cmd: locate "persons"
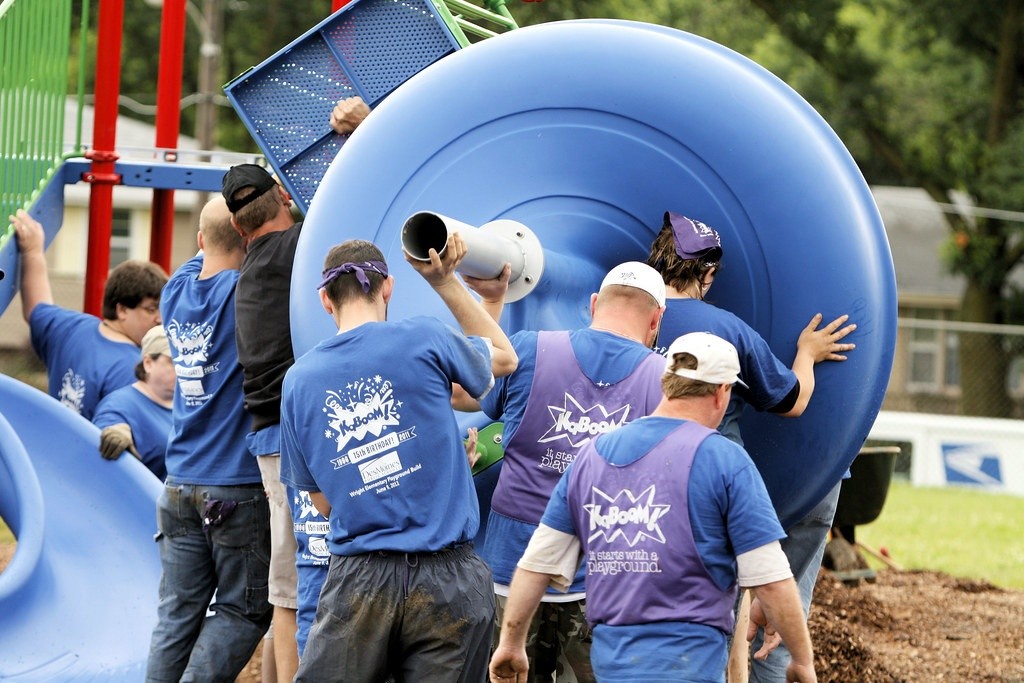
[745,478,842,683]
[294,423,485,670]
[451,260,677,683]
[650,210,857,459]
[272,231,520,683]
[145,197,275,683]
[91,325,176,488]
[489,331,817,683]
[10,207,173,429]
[220,96,371,683]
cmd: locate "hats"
[665,331,749,389]
[222,164,279,214]
[140,325,172,358]
[598,261,666,308]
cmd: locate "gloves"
[99,423,142,461]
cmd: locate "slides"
[0,374,165,683]
